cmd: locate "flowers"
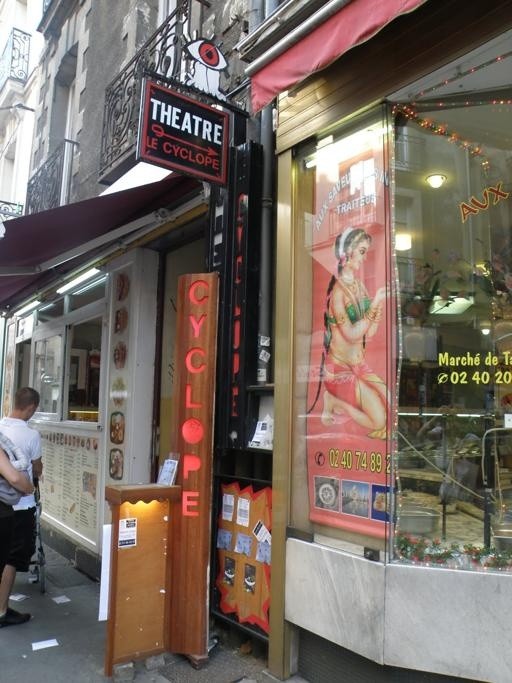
[398,530,512,570]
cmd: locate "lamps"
[405,291,477,316]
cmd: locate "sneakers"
[0,607,31,629]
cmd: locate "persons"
[417,405,482,504]
[307,228,396,431]
[0,387,43,627]
